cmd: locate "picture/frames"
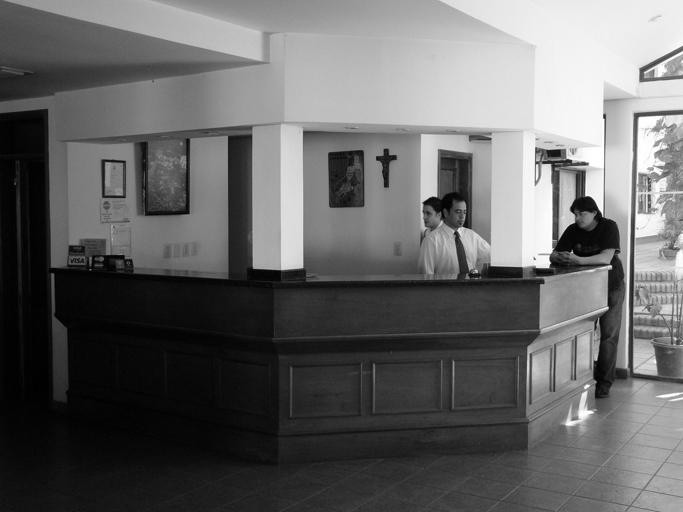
[101,159,126,198]
[141,139,190,215]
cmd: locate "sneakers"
[595,380,611,398]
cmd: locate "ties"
[454,230,470,274]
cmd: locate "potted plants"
[636,273,683,378]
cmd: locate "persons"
[417,192,491,274]
[421,195,443,241]
[549,196,626,398]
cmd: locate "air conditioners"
[543,148,581,162]
